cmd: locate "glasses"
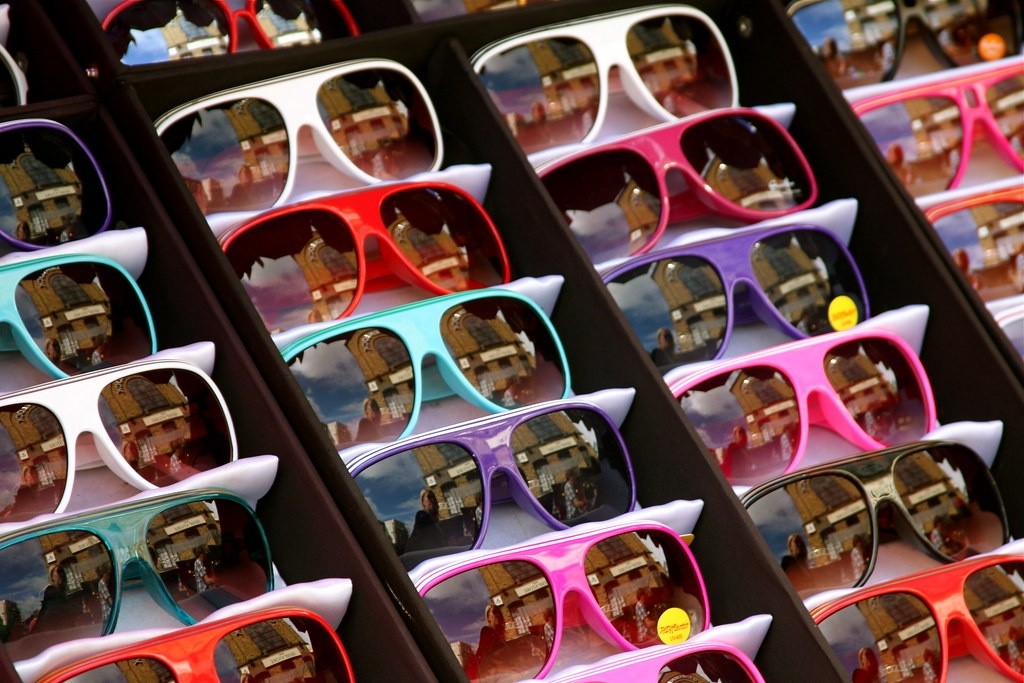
[153,57,444,214]
[0,40,31,111]
[0,251,158,380]
[0,486,274,662]
[409,518,710,683]
[1,119,112,248]
[34,606,356,682]
[217,182,510,337]
[271,288,571,451]
[344,399,638,573]
[550,638,766,683]
[99,0,362,65]
[0,359,240,521]
[810,556,1023,683]
[405,0,1024,598]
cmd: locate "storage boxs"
[0,0,1023,683]
